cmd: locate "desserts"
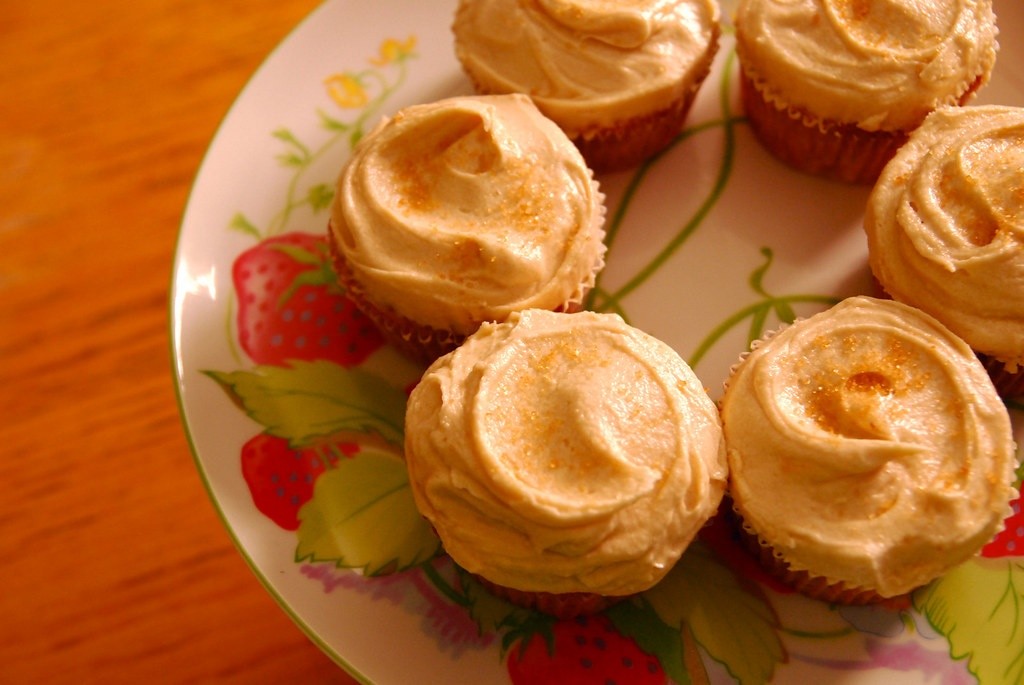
[324,0,1023,619]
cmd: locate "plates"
[167,0,1024,685]
